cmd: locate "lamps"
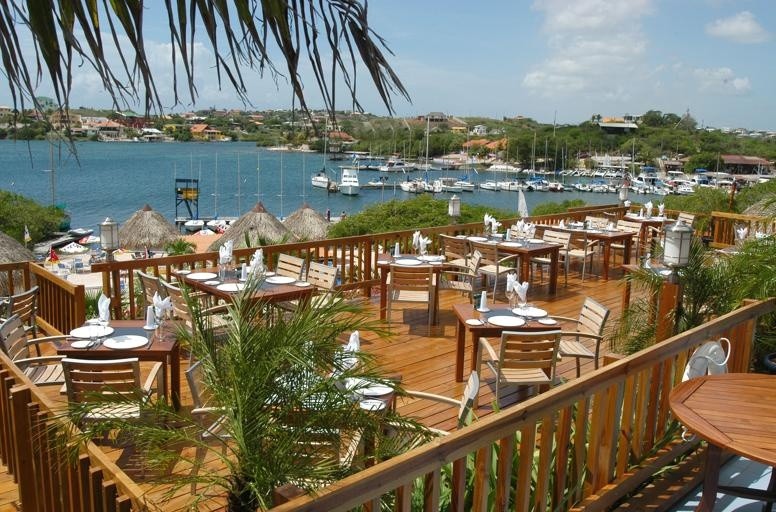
[449,195,460,225]
[97,217,119,262]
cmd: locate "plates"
[477,307,490,313]
[175,269,311,293]
[455,233,545,248]
[68,318,159,349]
[466,319,485,326]
[488,314,525,327]
[538,319,557,325]
[512,306,548,318]
[659,270,678,276]
[272,374,395,410]
[377,255,446,266]
[543,222,621,234]
[627,213,667,221]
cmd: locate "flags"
[47,248,59,265]
[22,224,32,242]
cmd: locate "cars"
[32,254,45,262]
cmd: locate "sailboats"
[184,150,306,233]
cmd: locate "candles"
[640,208,643,217]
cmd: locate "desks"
[670,372,776,512]
[452,303,561,382]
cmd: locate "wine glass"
[155,311,169,343]
[504,286,527,318]
[484,221,498,239]
[99,310,112,332]
[412,238,427,257]
[733,223,746,253]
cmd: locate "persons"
[326,177,331,197]
[339,211,347,221]
[327,209,330,222]
[143,245,147,259]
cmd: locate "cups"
[647,208,652,218]
[217,255,236,281]
[515,225,535,248]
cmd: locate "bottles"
[146,305,155,327]
[639,251,651,270]
[394,243,399,256]
[640,208,644,217]
[390,245,395,256]
[502,229,511,241]
[589,219,592,228]
[472,293,481,310]
[480,290,487,310]
[583,221,587,230]
[241,263,247,279]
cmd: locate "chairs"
[548,296,610,379]
[473,331,562,408]
[0,254,401,496]
[377,212,564,337]
[527,212,694,287]
[374,371,480,467]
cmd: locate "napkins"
[250,248,264,274]
[219,241,233,264]
[658,202,665,216]
[644,201,653,215]
